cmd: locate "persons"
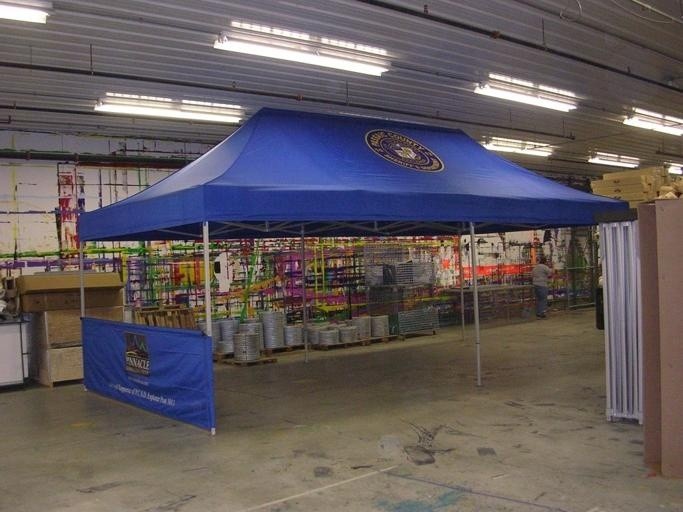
[532,255,552,320]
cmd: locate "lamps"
[587,149,642,168]
[622,106,682,139]
[1,0,54,26]
[472,71,584,112]
[478,135,560,159]
[665,162,681,176]
[93,91,244,126]
[213,20,398,79]
